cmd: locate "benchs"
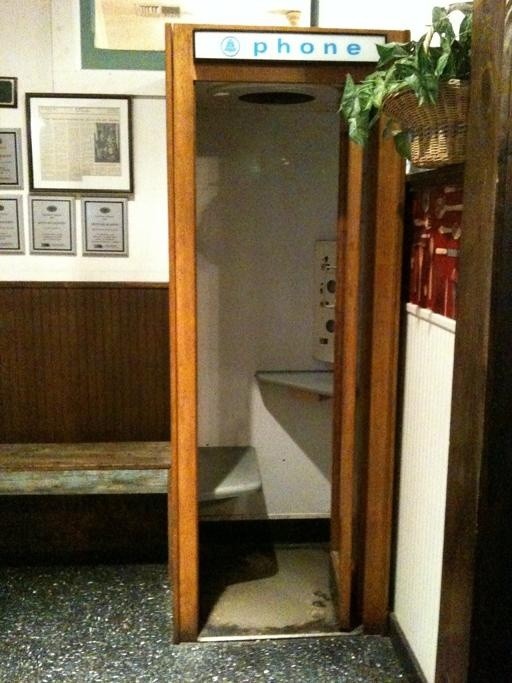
[0,282,170,497]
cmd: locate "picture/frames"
[24,92,134,194]
[0,76,18,108]
[50,0,319,97]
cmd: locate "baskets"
[379,5,472,169]
